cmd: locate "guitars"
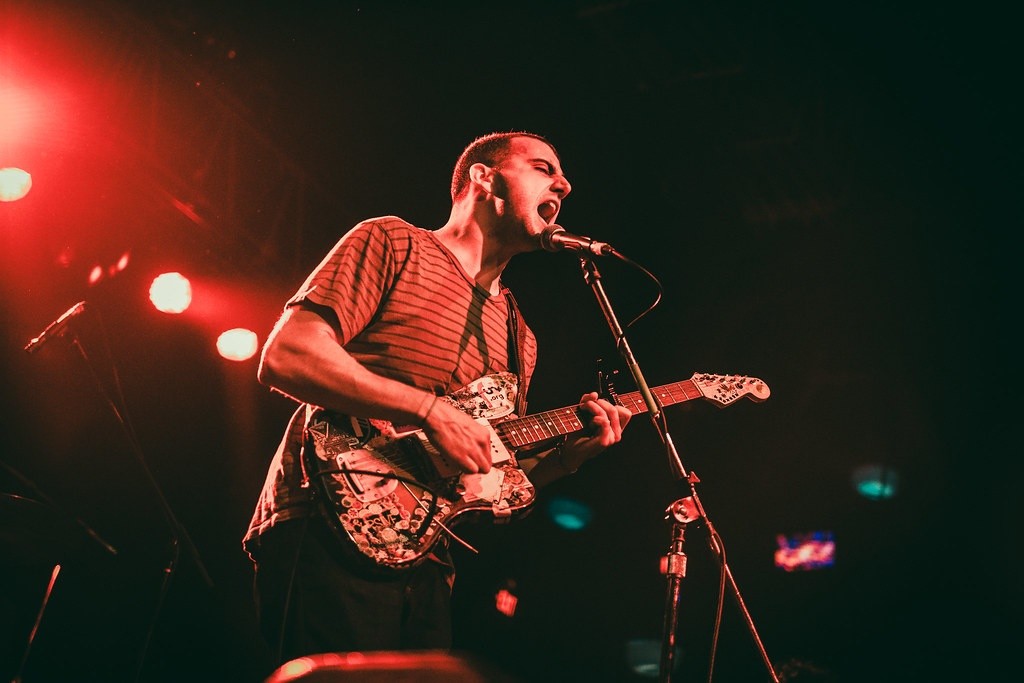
[301,368,771,579]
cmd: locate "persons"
[241,128,632,682]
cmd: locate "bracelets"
[413,390,438,428]
[555,440,578,479]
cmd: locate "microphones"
[26,302,88,355]
[540,224,615,256]
